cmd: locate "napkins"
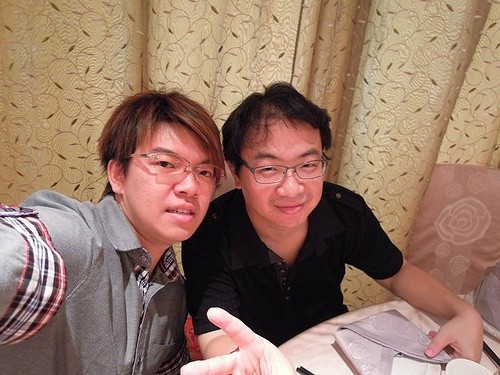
[333,310,452,375]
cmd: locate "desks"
[278,294,500,375]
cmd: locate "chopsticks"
[482,342,500,366]
[297,366,316,375]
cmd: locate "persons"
[179,82,486,375]
[0,89,224,375]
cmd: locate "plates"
[392,356,442,375]
[464,290,500,340]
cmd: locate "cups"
[445,358,495,375]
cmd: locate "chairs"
[406,164,500,296]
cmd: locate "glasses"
[123,151,227,186]
[242,151,331,183]
